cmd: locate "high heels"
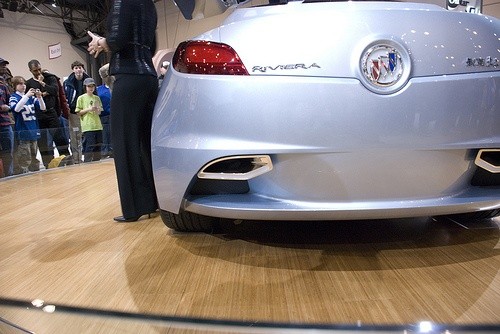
[114,209,156,222]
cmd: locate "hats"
[161,61,170,68]
[83,78,96,85]
[0,58,9,64]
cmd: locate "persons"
[86,0,159,222]
[0,59,117,176]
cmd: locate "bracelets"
[97,35,102,51]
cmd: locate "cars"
[150,0,500,234]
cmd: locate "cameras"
[33,89,39,95]
